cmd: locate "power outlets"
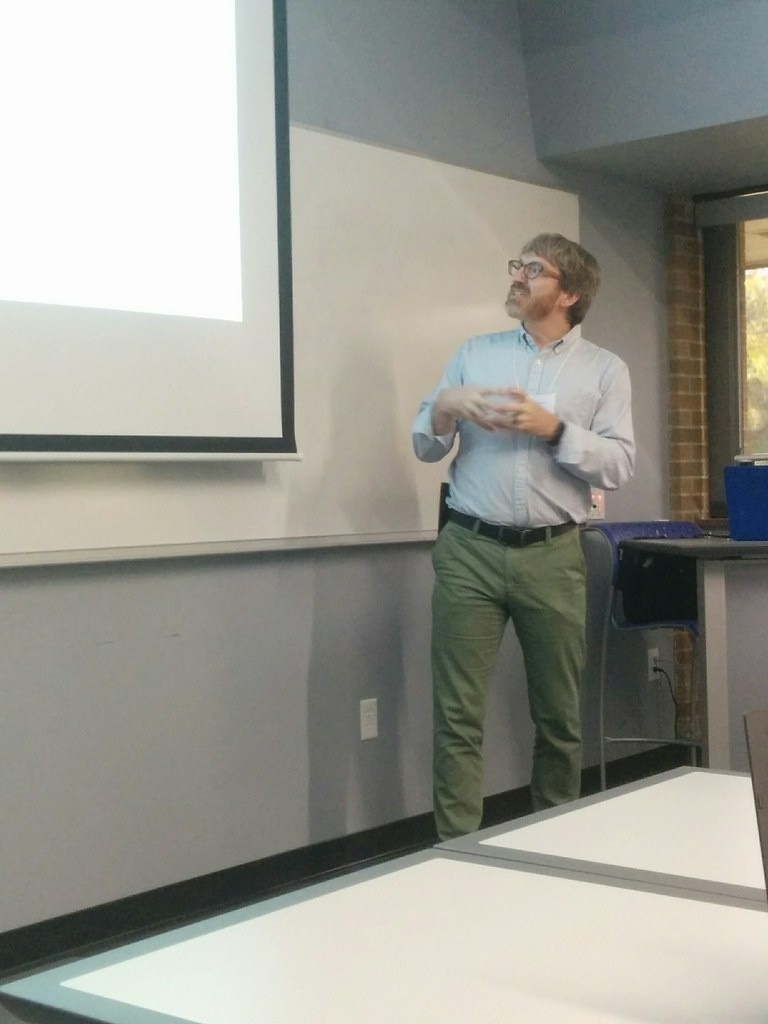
[647,647,660,682]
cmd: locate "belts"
[447,508,575,547]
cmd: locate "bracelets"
[547,422,564,446]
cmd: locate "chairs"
[581,520,701,791]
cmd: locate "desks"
[619,536,768,891]
[0,765,768,1024]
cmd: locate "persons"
[411,232,638,841]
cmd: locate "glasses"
[508,260,561,279]
